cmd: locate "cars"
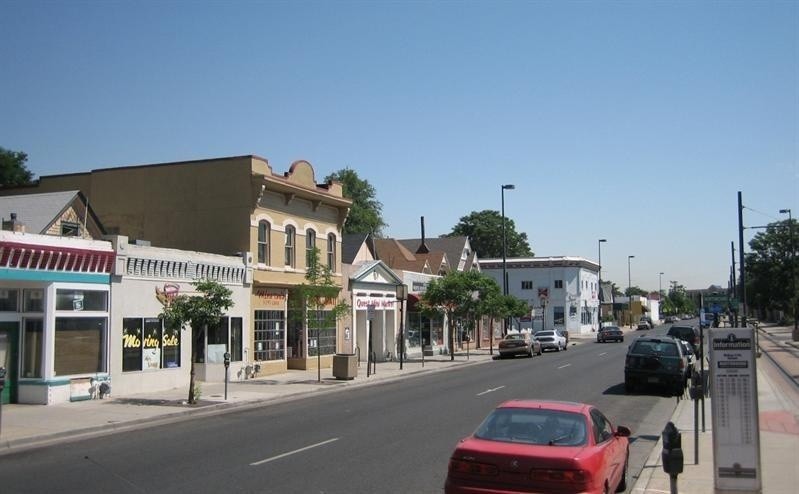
[665,316,674,324]
[596,327,624,343]
[659,272,663,303]
[443,400,631,494]
[534,330,567,352]
[626,331,697,395]
[498,333,542,358]
[639,316,654,329]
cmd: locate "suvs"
[668,325,701,358]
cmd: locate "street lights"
[597,238,606,329]
[742,206,795,292]
[501,183,515,332]
[628,255,634,328]
